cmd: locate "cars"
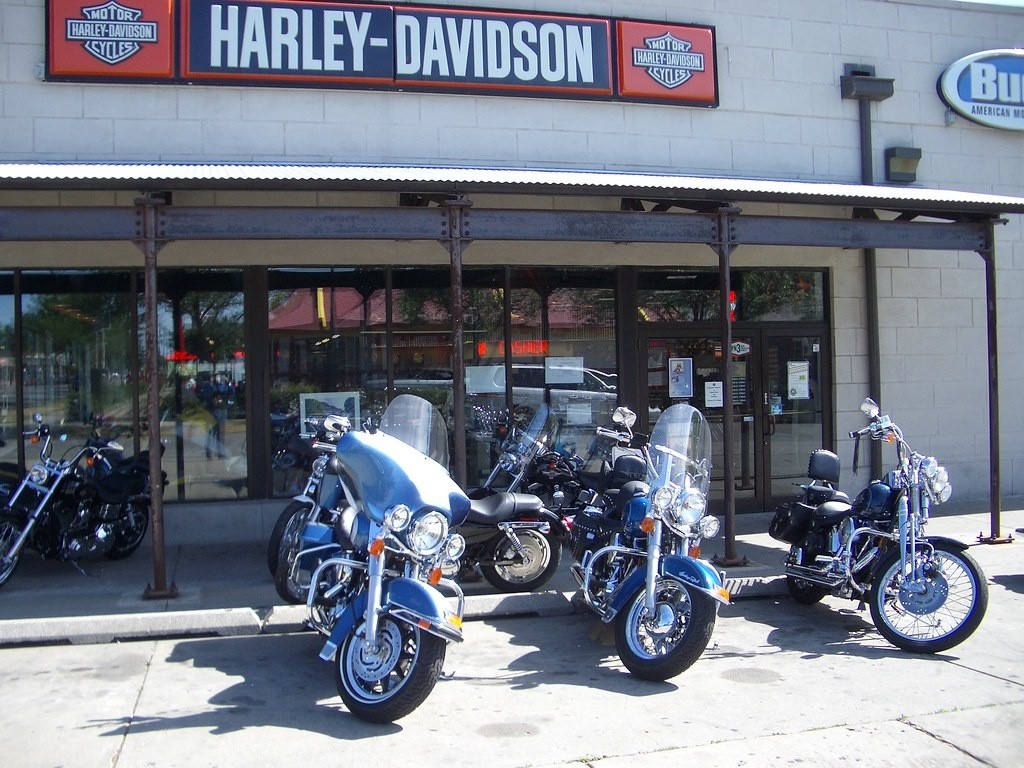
[503,364,665,429]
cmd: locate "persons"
[206,374,230,457]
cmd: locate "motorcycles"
[287,393,474,726]
[0,412,172,590]
[267,399,733,682]
[768,398,990,654]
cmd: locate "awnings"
[0,162,1024,218]
